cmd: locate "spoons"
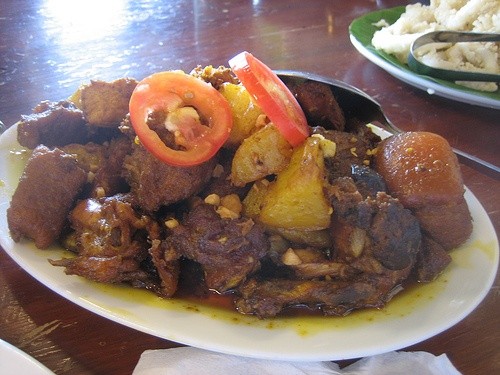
[273,69,499,180]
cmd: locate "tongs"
[406,30,500,80]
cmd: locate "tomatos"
[128,51,309,167]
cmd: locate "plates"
[2,112,499,362]
[348,3,500,110]
